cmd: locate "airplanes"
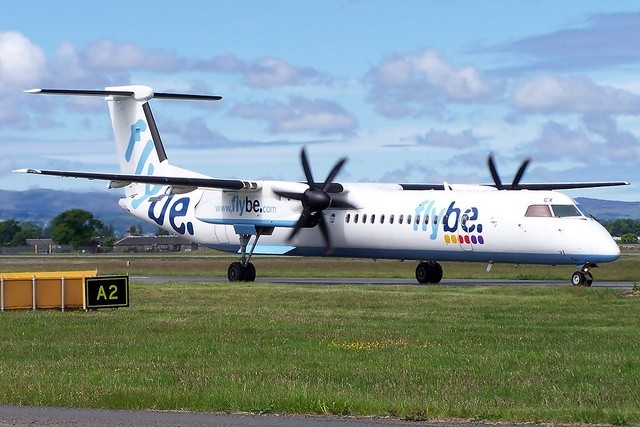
[11,85,630,285]
